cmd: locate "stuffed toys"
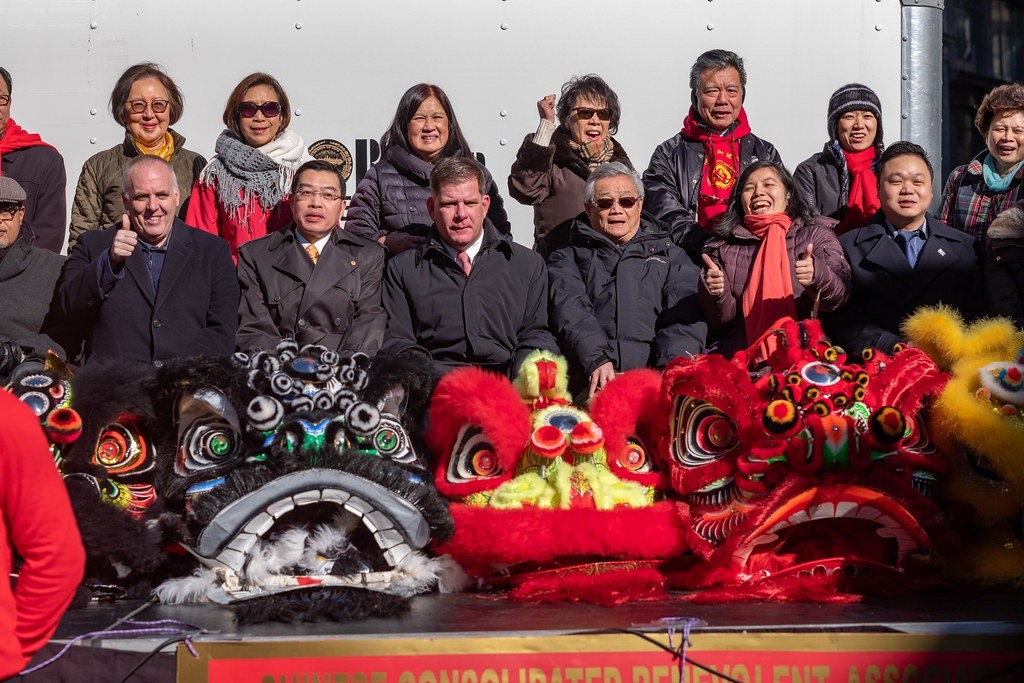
[0,307,1024,625]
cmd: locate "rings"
[544,96,549,100]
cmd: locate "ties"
[304,244,319,266]
[457,251,472,276]
[898,229,918,269]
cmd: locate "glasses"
[238,101,282,118]
[126,99,170,113]
[0,206,20,221]
[291,189,344,200]
[591,195,641,209]
[0,95,11,105]
[568,106,614,121]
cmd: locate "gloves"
[0,339,22,374]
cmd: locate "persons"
[0,176,85,387]
[345,84,512,259]
[381,157,561,380]
[699,160,852,385]
[67,62,208,256]
[51,154,241,375]
[185,73,317,266]
[933,85,1023,332]
[508,75,639,263]
[0,385,87,683]
[235,161,389,361]
[640,49,791,267]
[0,68,67,253]
[547,162,709,414]
[819,141,986,366]
[793,83,884,239]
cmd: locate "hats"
[0,176,26,203]
[827,83,883,143]
